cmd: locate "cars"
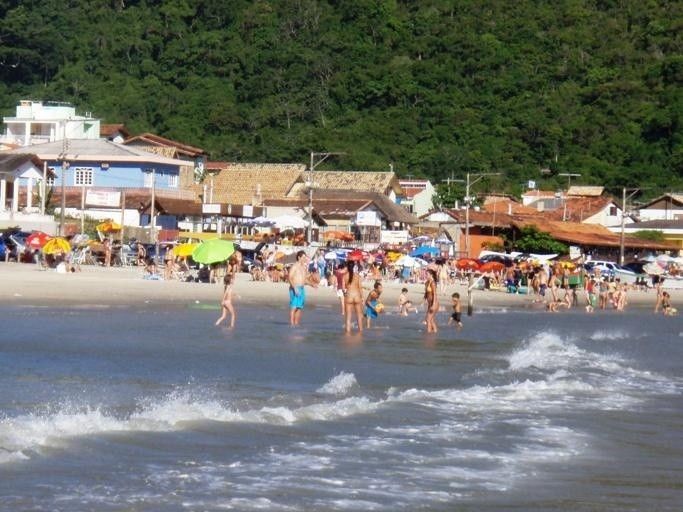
[584,261,645,276]
[479,254,505,263]
[159,237,205,252]
[111,245,165,266]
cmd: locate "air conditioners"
[101,162,109,168]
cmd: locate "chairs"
[74,251,85,264]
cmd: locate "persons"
[286,250,308,327]
[420,268,440,333]
[0,224,682,317]
[339,259,364,335]
[364,281,383,329]
[214,274,235,329]
[446,292,463,329]
